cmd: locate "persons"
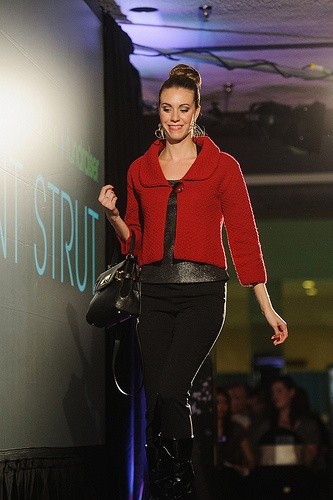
[98,64,290,500]
[215,379,333,500]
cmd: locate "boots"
[147,462,194,500]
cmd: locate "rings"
[104,196,109,198]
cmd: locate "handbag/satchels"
[85,229,143,330]
